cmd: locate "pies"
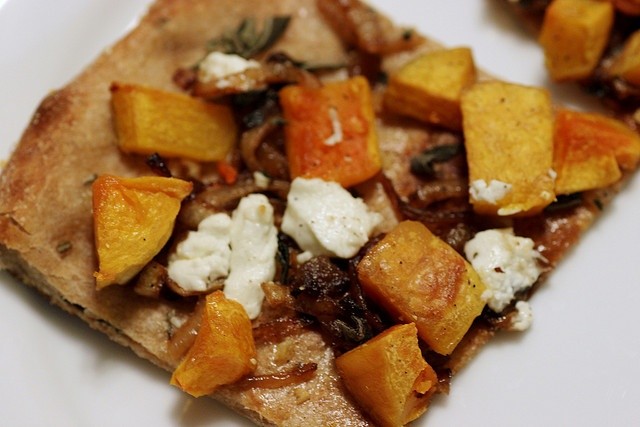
[500,0,640,126]
[0,1,639,425]
